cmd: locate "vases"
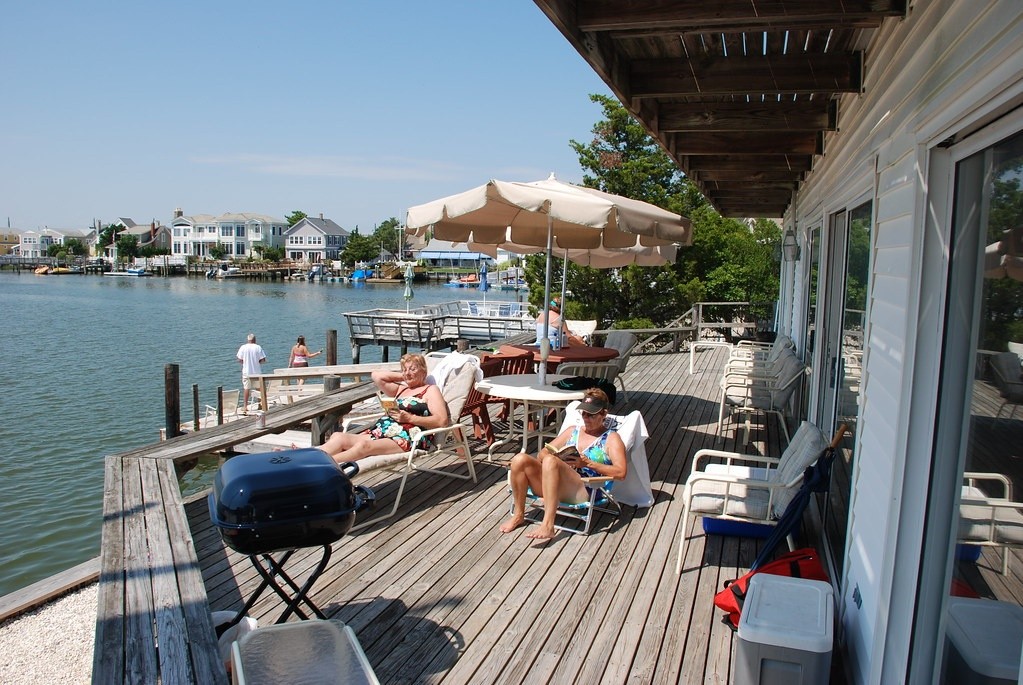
[755,331,777,349]
[731,336,756,346]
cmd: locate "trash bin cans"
[734,572,834,685]
[755,332,777,347]
[942,595,1023,685]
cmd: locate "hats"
[575,396,608,415]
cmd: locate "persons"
[288,334,322,397]
[499,388,626,538]
[272,353,449,466]
[236,334,268,413]
[535,297,584,347]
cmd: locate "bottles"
[553,333,560,350]
[562,332,568,347]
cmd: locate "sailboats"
[443,250,464,288]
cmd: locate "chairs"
[203,389,240,428]
[338,321,656,536]
[957,473,1023,576]
[468,302,535,329]
[717,333,806,445]
[675,421,831,578]
[988,341,1023,431]
[841,345,864,415]
[250,379,284,412]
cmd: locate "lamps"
[782,225,802,263]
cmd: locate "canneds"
[256,411,265,429]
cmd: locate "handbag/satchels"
[713,548,828,631]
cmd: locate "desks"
[499,344,620,432]
[473,372,595,462]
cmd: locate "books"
[376,390,397,414]
[545,442,581,464]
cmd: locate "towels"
[558,400,656,509]
[426,350,484,394]
[552,376,617,406]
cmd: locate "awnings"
[420,246,480,267]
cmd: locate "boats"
[343,269,373,282]
[53,266,70,273]
[35,265,49,274]
[303,263,332,281]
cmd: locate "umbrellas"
[406,174,699,382]
[479,262,489,307]
[403,263,414,314]
[749,423,847,573]
